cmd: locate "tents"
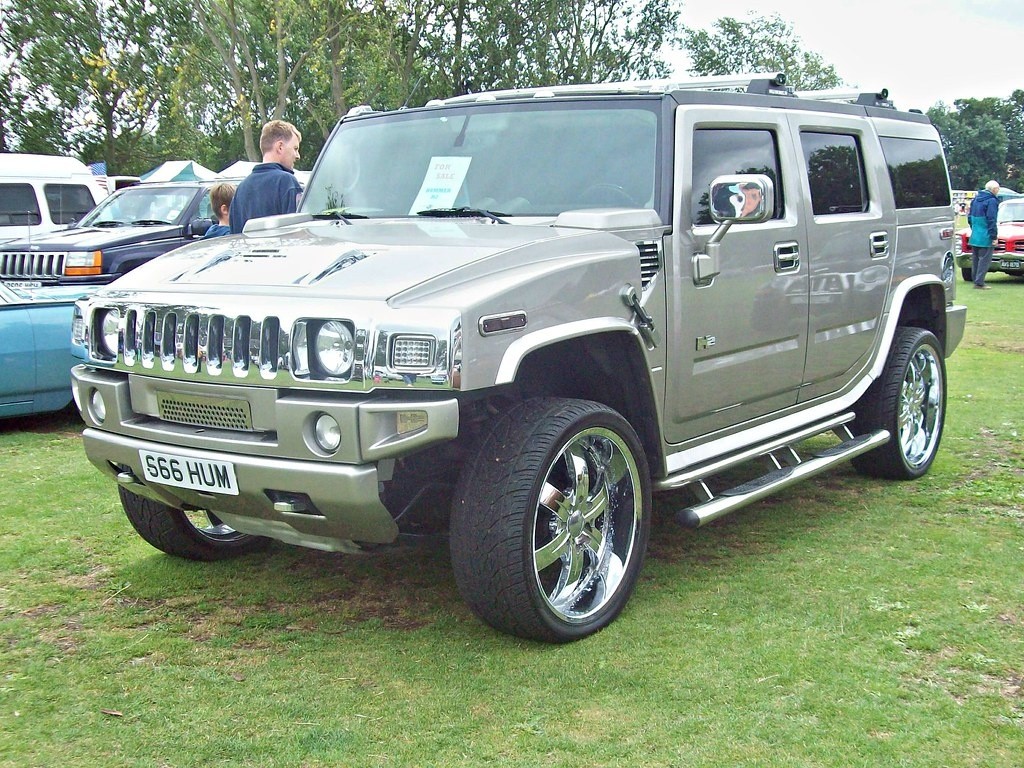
[219,159,312,183]
[139,158,225,182]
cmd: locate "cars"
[954,197,1024,283]
[2,175,250,425]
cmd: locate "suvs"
[72,64,971,644]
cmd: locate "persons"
[967,179,1000,289]
[198,183,235,239]
[227,119,303,233]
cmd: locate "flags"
[87,163,108,189]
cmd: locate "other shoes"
[973,284,992,290]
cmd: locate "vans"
[0,150,114,240]
